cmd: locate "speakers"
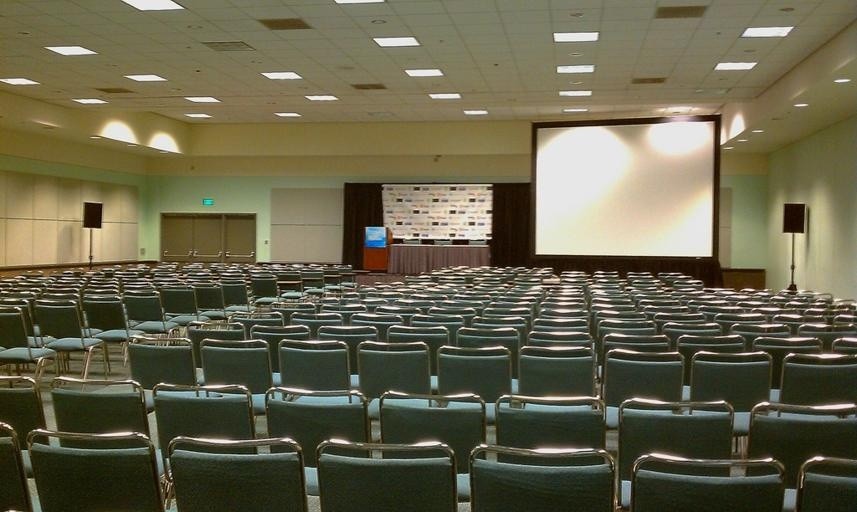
[782,202,805,234]
[82,202,103,229]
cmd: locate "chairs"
[421,239,434,245]
[452,239,469,245]
[393,239,403,244]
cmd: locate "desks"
[387,244,492,273]
